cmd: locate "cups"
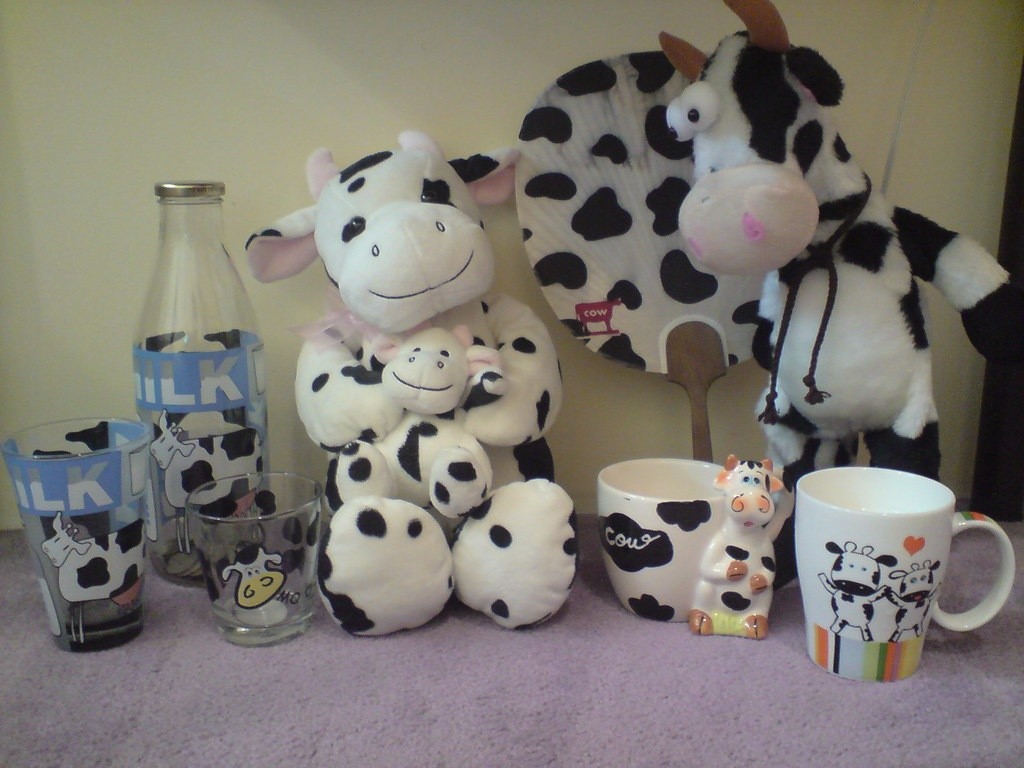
[796,465,1017,682]
[0,416,155,654]
[184,471,323,647]
[595,456,795,624]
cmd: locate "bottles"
[129,178,268,590]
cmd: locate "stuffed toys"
[657,2,1023,592]
[333,327,507,521]
[238,127,582,640]
